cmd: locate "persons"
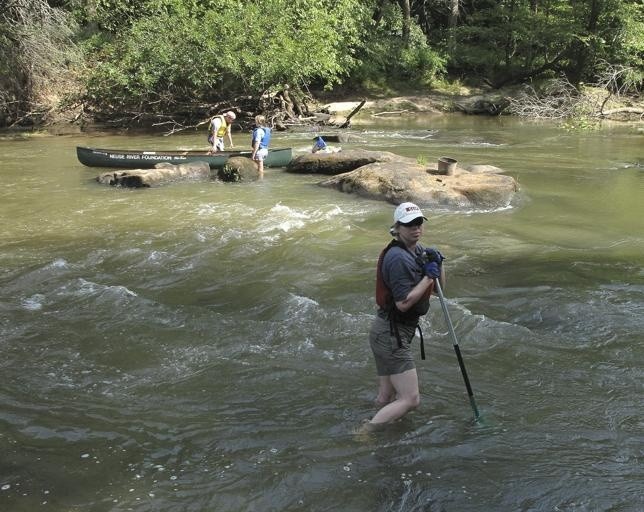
[250,114,272,182]
[353,202,447,450]
[207,111,236,154]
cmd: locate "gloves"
[423,261,440,278]
[423,247,443,265]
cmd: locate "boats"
[74,142,292,174]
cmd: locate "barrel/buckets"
[438,156,458,175]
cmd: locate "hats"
[394,202,428,224]
[223,111,236,120]
